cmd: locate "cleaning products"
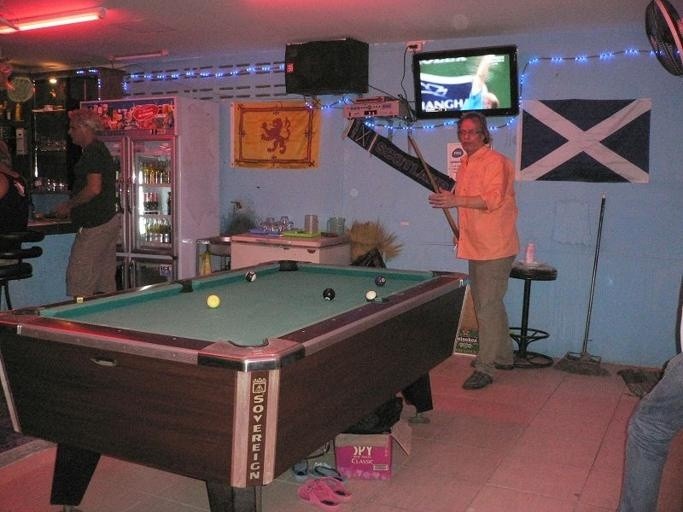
[525,240,536,265]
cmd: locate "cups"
[327,216,345,235]
[263,215,293,233]
[304,214,318,234]
[37,135,66,152]
[43,178,66,194]
[42,105,53,112]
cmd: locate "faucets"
[229,200,243,217]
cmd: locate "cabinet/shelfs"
[226,224,354,271]
[0,66,128,225]
[193,231,235,278]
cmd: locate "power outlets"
[405,39,424,53]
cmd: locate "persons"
[460,55,498,110]
[614,302,683,512]
[428,112,519,390]
[0,139,28,267]
[54,107,120,299]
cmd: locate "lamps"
[0,5,105,35]
[109,47,168,63]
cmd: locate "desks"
[0,258,476,510]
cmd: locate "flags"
[520,98,652,183]
[231,99,320,169]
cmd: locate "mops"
[551,194,616,378]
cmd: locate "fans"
[643,0,682,78]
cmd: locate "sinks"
[208,235,232,258]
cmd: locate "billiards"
[207,295,220,309]
[323,288,335,301]
[245,271,257,281]
[365,290,376,302]
[375,276,386,286]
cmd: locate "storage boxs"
[325,388,419,483]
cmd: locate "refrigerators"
[80,96,223,292]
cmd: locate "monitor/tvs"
[412,44,520,120]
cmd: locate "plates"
[518,260,546,268]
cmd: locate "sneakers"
[470,359,514,370]
[462,371,493,389]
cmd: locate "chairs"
[0,225,48,311]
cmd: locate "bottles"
[112,156,122,214]
[526,242,535,264]
[137,158,172,244]
[14,102,21,121]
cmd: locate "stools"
[500,262,559,369]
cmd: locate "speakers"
[70,67,127,101]
[284,39,369,95]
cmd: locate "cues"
[407,135,459,241]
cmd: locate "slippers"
[292,461,353,511]
